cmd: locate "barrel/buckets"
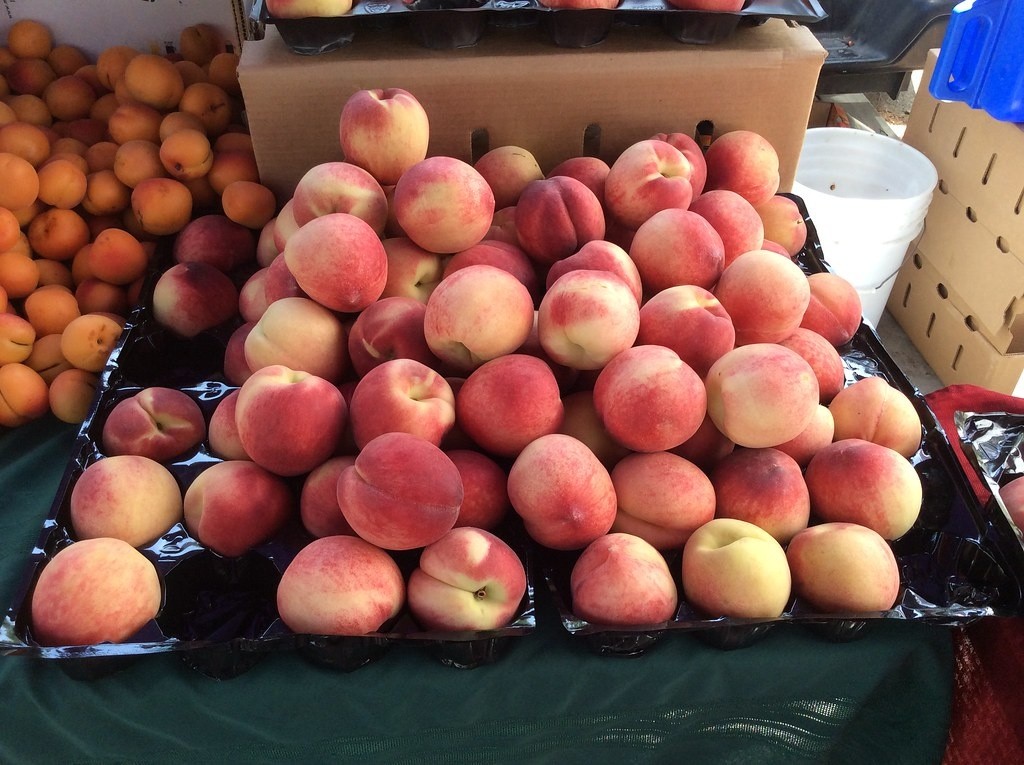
[789,124,939,330]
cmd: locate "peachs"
[0,4,923,649]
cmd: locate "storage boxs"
[236,16,830,220]
[885,48,1024,399]
